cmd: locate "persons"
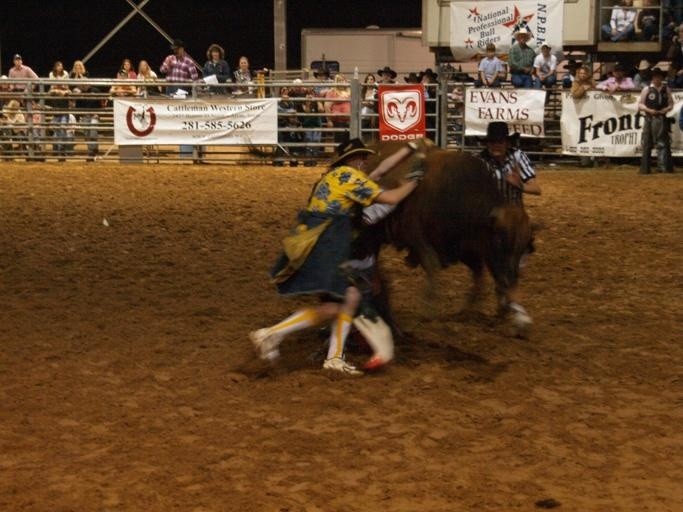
[268,64,481,168]
[471,120,542,332]
[467,0,682,173]
[248,135,424,379]
[1,42,258,163]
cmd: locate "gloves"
[406,152,425,179]
[406,137,436,149]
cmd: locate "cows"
[306,139,550,324]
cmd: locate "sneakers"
[321,355,363,379]
[249,327,280,370]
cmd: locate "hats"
[648,67,667,77]
[474,121,520,142]
[377,67,437,83]
[328,138,375,168]
[170,39,184,48]
[514,28,532,41]
[313,68,328,78]
[4,100,25,109]
[635,60,650,70]
[539,43,551,49]
